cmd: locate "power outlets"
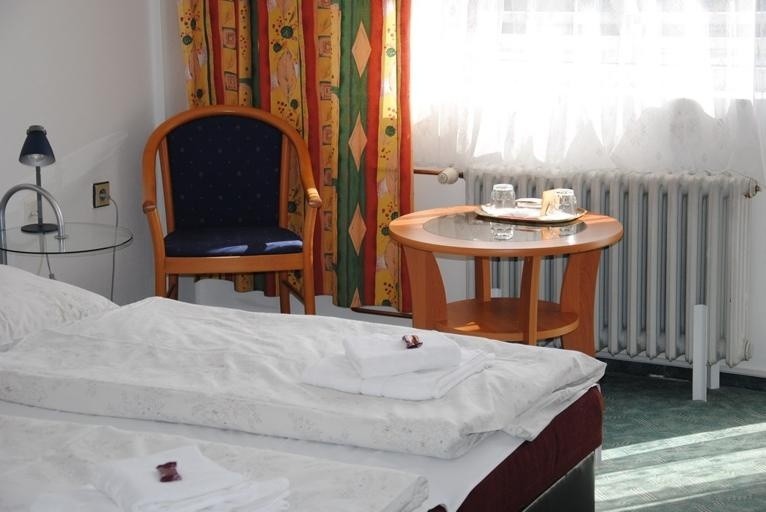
[93,181,110,208]
[22,198,38,222]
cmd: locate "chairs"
[142,105,323,316]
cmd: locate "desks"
[0,221,133,281]
[388,206,623,359]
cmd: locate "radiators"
[464,164,761,368]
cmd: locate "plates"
[473,202,587,224]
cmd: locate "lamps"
[18,125,60,235]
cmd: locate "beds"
[0,263,606,512]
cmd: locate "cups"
[558,220,576,240]
[488,220,516,241]
[549,187,577,218]
[490,183,516,212]
